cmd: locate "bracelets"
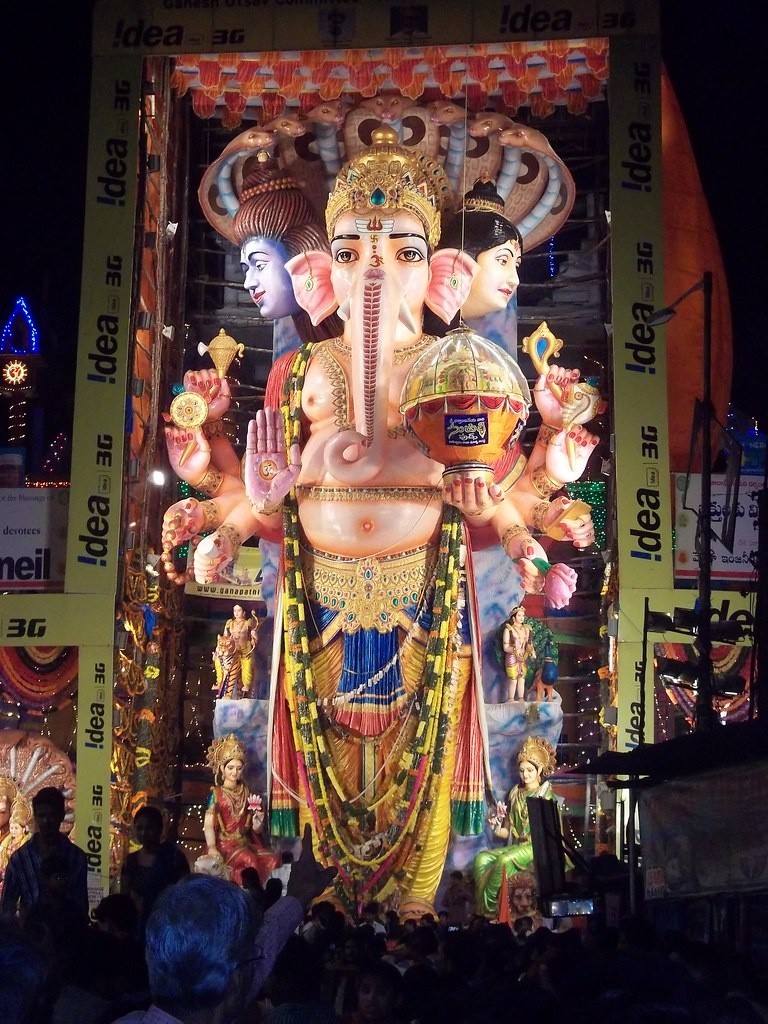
[197,419,225,439]
[531,501,552,533]
[500,524,529,551]
[534,421,560,447]
[217,522,242,553]
[191,466,225,497]
[531,466,562,497]
[249,501,284,519]
[201,499,222,534]
[208,844,216,851]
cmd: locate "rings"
[533,388,547,392]
[549,440,562,447]
[219,394,231,398]
[200,449,211,453]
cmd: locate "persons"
[0,893,152,1024]
[112,823,338,1024]
[0,786,91,938]
[120,806,191,941]
[162,124,607,925]
[1,777,33,888]
[226,850,768,1024]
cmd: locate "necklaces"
[519,790,536,817]
[330,334,435,365]
[514,624,524,630]
